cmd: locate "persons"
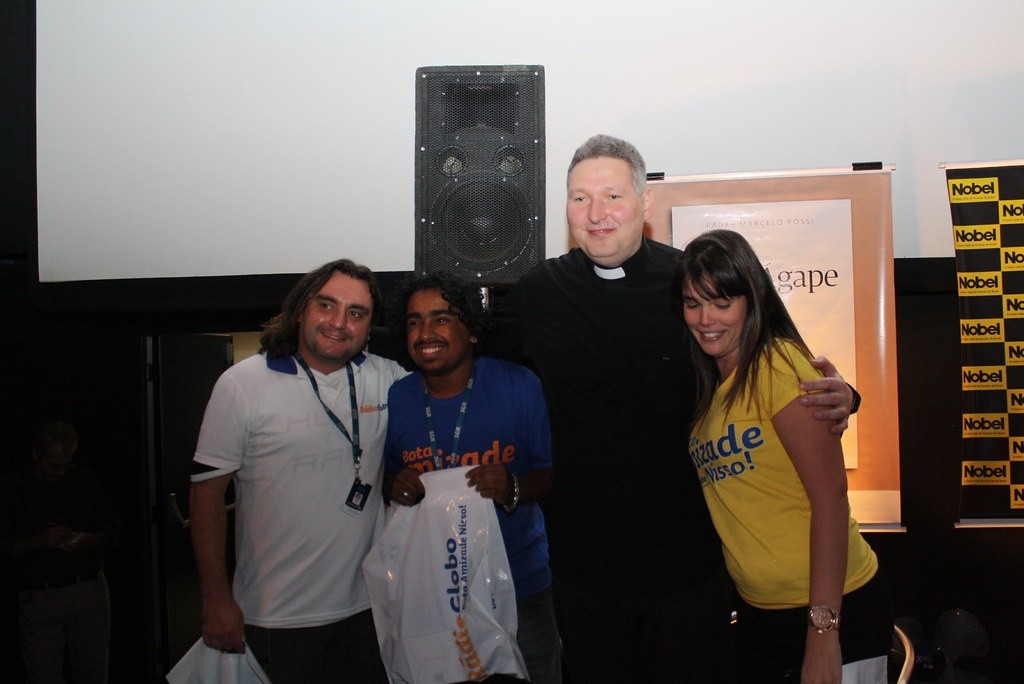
[485,133,862,684]
[0,422,121,684]
[680,229,896,684]
[382,272,564,684]
[191,258,413,684]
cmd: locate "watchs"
[809,605,840,633]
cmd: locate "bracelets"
[502,473,520,513]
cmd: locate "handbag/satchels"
[362,463,531,684]
[165,635,272,684]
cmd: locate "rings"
[402,492,408,498]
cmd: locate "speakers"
[414,64,546,284]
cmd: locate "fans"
[890,596,1010,684]
